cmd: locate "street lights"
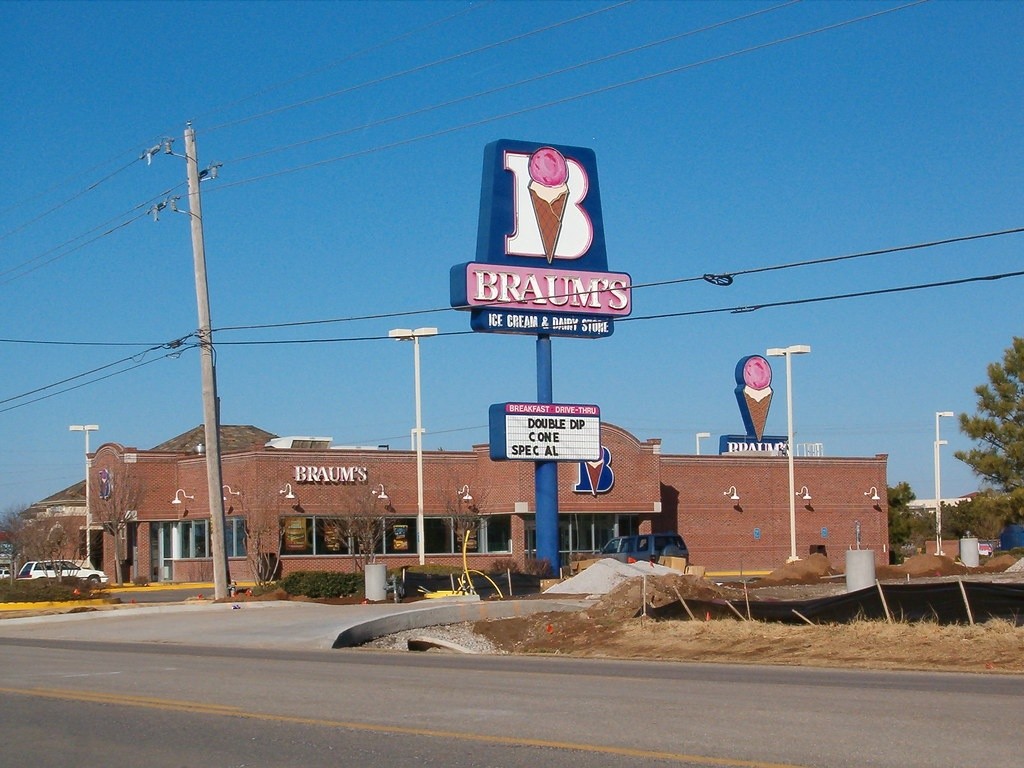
[932,440,949,556]
[387,327,439,566]
[765,344,811,565]
[70,425,99,570]
[695,432,711,455]
[410,428,426,451]
[936,410,954,557]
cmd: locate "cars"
[0,569,11,580]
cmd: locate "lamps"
[280,483,295,498]
[372,484,388,499]
[724,486,739,500]
[222,485,239,501]
[458,485,473,499]
[172,489,193,504]
[864,487,880,499]
[796,486,812,499]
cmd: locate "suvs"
[15,560,109,585]
[591,533,691,566]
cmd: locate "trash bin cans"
[114,560,130,583]
[256,552,278,581]
[810,545,826,557]
